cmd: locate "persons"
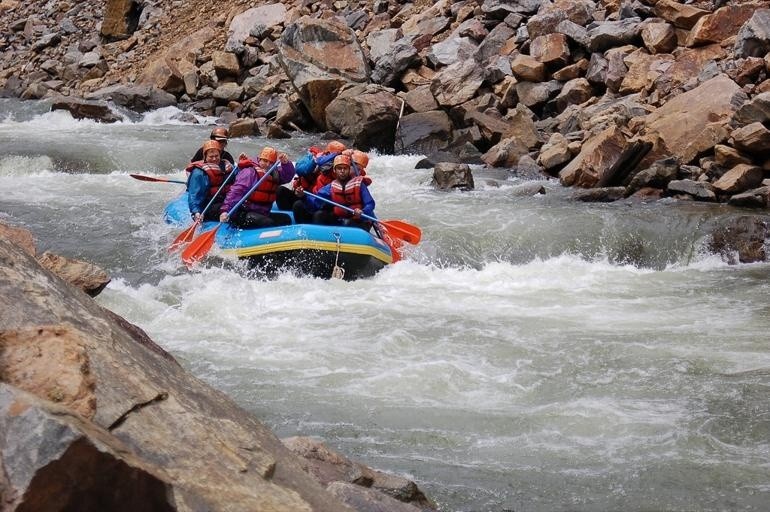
[187,128,377,231]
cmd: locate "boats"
[163,190,394,284]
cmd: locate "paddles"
[182,160,281,266]
[168,168,237,246]
[302,190,420,244]
[350,157,401,264]
[130,174,188,185]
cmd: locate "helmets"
[351,151,369,168]
[258,146,277,163]
[333,154,351,169]
[210,127,229,138]
[328,140,345,154]
[202,141,221,158]
